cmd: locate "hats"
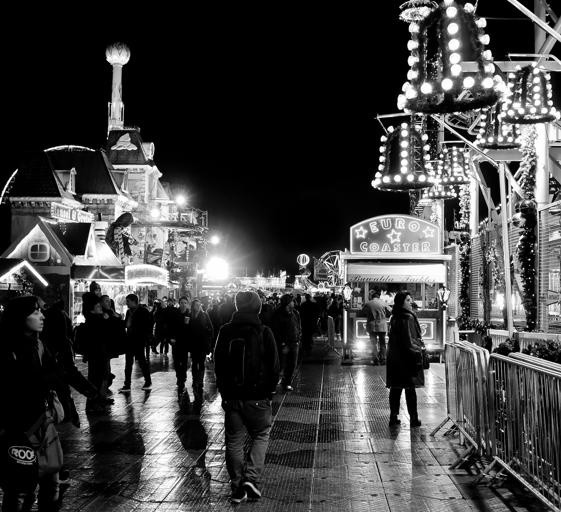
[236,292,262,314]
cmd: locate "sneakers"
[231,480,261,502]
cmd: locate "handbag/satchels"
[29,412,63,475]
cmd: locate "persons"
[385,291,431,429]
[39,281,237,425]
[1,294,66,511]
[213,290,282,504]
[238,282,347,392]
[362,292,393,366]
